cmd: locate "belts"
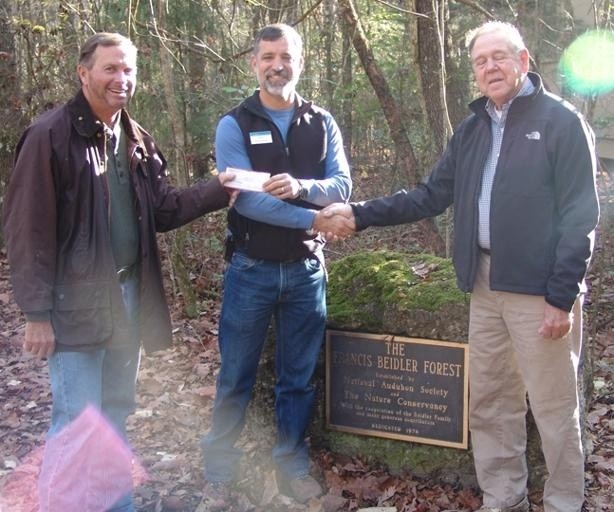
[116,262,140,284]
[477,245,490,256]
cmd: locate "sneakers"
[287,474,324,503]
[193,482,233,512]
[473,496,530,512]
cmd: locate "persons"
[200,23,354,512]
[4,32,240,512]
[318,19,600,512]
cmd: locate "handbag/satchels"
[223,241,235,262]
